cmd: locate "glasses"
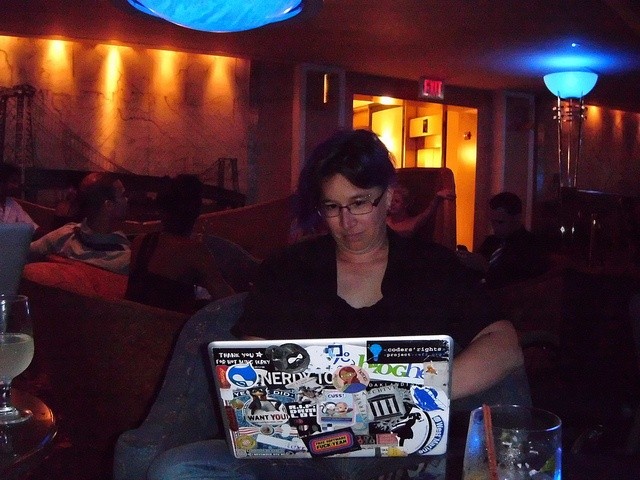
[314,187,387,218]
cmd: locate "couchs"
[21,249,547,447]
[8,193,293,269]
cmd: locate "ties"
[481,242,506,279]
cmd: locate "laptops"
[208,334,454,461]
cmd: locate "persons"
[458,192,550,279]
[29,172,146,305]
[145,129,524,480]
[1,162,40,235]
[385,184,456,241]
[131,173,233,313]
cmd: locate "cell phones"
[456,243,469,254]
